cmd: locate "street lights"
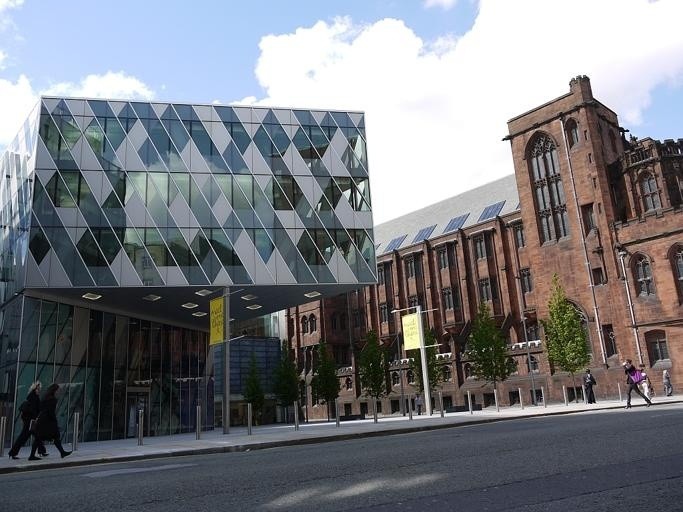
[390,305,432,415]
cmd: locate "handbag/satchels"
[28,420,39,432]
[627,368,644,384]
[586,378,597,386]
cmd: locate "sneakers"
[28,456,41,460]
[61,450,73,458]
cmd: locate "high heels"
[624,404,631,409]
[9,455,19,459]
[39,453,49,456]
[647,402,653,407]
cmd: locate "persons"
[662,369,673,396]
[8,380,50,460]
[415,394,423,415]
[582,368,597,404]
[639,367,652,400]
[28,382,73,461]
[622,358,652,409]
